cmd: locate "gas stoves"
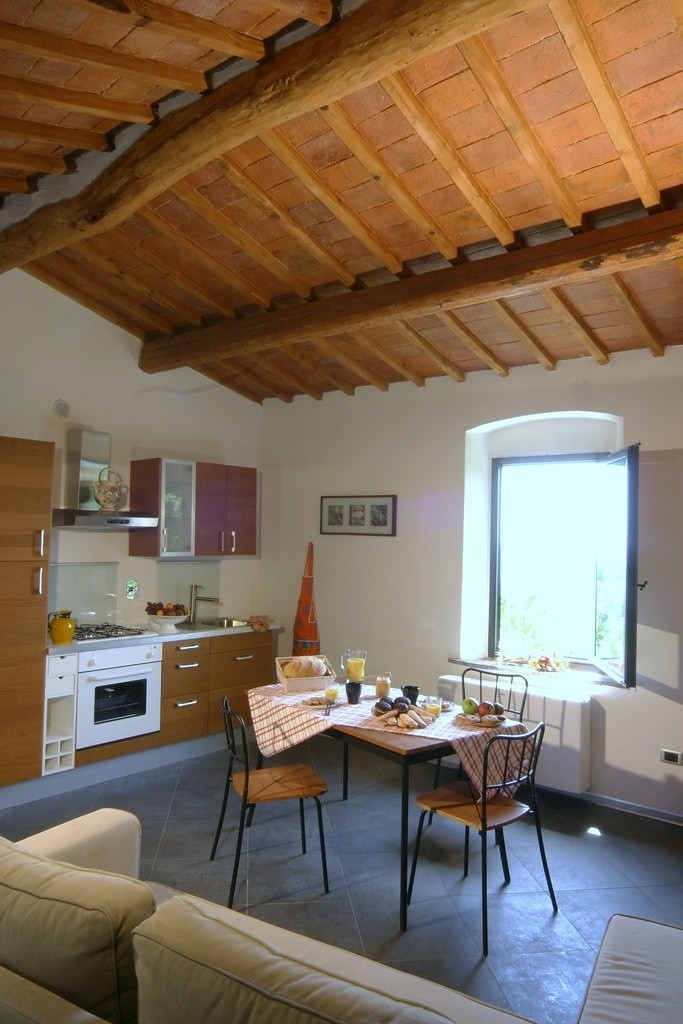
[66,621,164,653]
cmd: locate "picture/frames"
[321,494,397,536]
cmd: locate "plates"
[300,699,337,708]
[421,703,457,712]
[459,713,505,726]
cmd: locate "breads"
[374,696,436,728]
[283,656,327,677]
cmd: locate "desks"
[246,681,543,931]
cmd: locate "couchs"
[0,809,683,1024]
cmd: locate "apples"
[157,603,189,615]
[463,698,504,716]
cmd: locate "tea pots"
[91,468,128,513]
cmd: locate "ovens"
[75,648,161,750]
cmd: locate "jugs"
[48,609,76,644]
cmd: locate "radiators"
[436,675,591,795]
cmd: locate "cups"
[324,682,338,701]
[375,674,390,698]
[401,685,420,706]
[340,649,367,683]
[346,680,362,704]
[425,696,443,718]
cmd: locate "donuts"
[456,713,499,724]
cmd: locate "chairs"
[209,696,330,909]
[409,722,557,957]
[427,668,528,827]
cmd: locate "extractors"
[51,427,160,530]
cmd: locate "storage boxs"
[275,656,336,694]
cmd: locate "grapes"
[146,601,164,614]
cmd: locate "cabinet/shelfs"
[130,458,260,557]
[42,654,77,777]
[160,632,278,747]
[0,437,58,788]
